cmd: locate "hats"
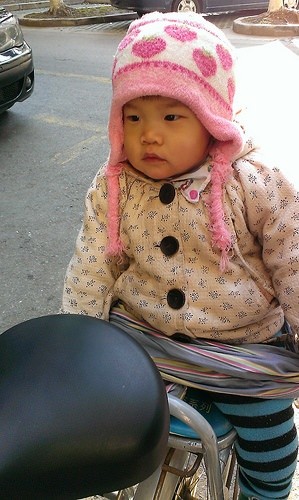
[108,8,243,272]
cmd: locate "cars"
[0,4,36,115]
[109,0,299,17]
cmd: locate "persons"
[58,11,299,500]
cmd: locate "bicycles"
[0,310,243,500]
